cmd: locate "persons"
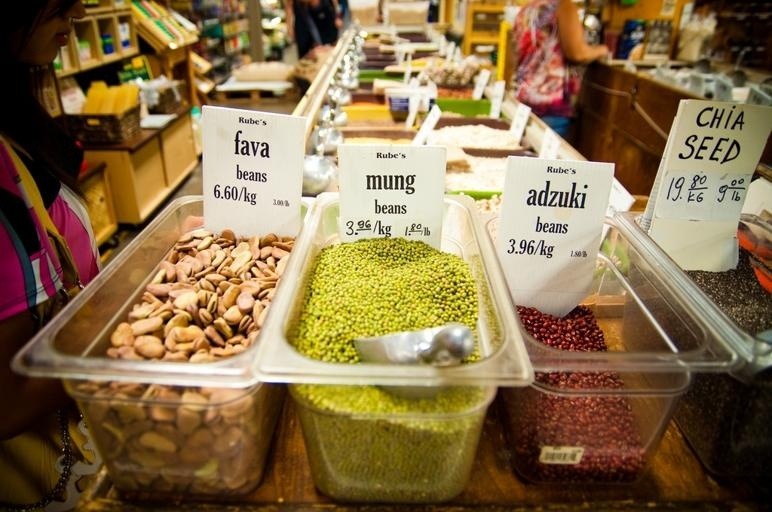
[677,0,719,62]
[2,1,110,512]
[511,1,610,136]
[292,1,348,58]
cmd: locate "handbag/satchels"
[0,135,111,510]
[512,36,574,114]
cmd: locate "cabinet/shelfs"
[35,1,293,256]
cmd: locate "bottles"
[679,13,717,63]
[615,16,645,63]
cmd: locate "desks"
[77,290,757,511]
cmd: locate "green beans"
[285,238,503,504]
[497,304,648,486]
[76,229,296,497]
